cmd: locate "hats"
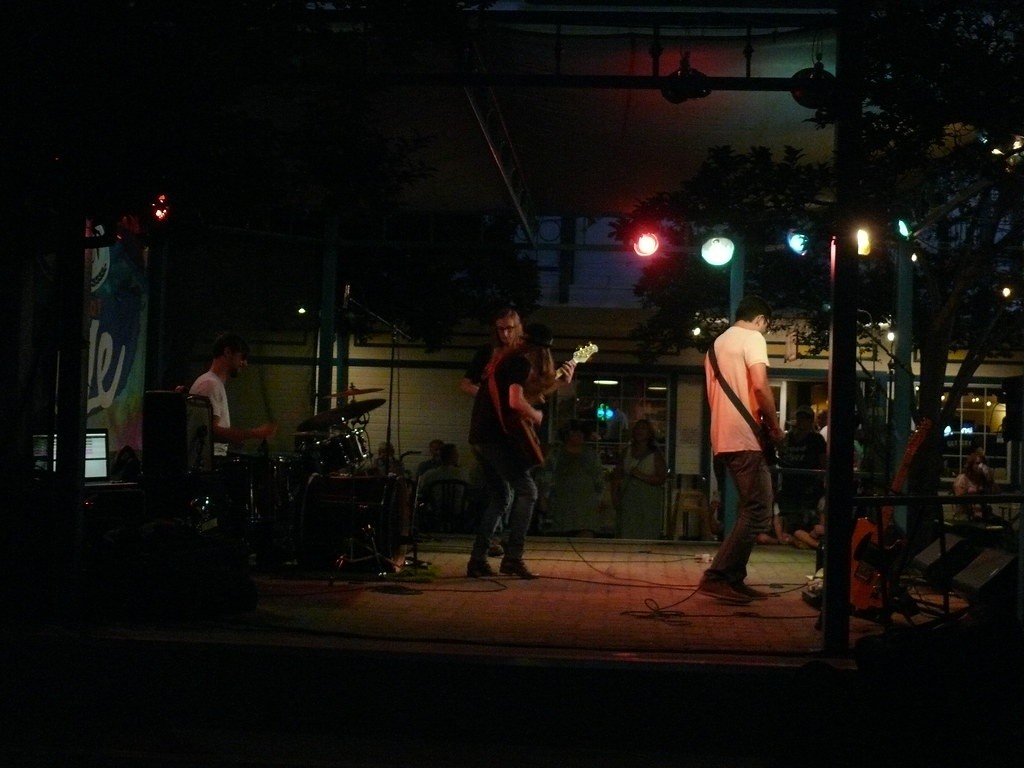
[518,321,555,348]
[793,405,814,418]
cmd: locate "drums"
[293,473,410,575]
[294,431,369,473]
[233,450,299,529]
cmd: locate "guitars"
[517,412,544,467]
[850,415,934,620]
[749,379,776,458]
[555,341,599,381]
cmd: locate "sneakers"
[697,576,769,603]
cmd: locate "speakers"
[142,389,216,479]
[911,532,1019,617]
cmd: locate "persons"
[188,330,278,469]
[459,307,575,581]
[698,296,786,602]
[953,450,995,527]
[532,397,668,541]
[361,440,468,535]
[708,407,867,550]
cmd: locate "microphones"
[344,283,351,309]
[197,424,209,439]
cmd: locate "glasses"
[497,325,515,332]
[763,316,772,334]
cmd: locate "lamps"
[789,66,839,109]
[660,67,712,105]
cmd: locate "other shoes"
[794,538,812,549]
[485,538,509,556]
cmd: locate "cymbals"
[296,398,387,431]
[322,387,384,400]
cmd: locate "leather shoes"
[466,560,499,582]
[500,556,540,580]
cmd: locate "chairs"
[408,462,489,568]
